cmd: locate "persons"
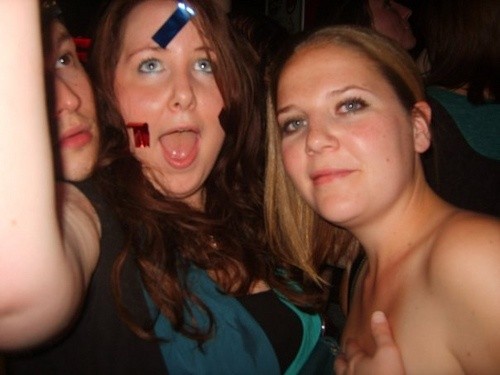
[304,0,497,214]
[263,24,499,374]
[0,0,334,375]
[40,8,103,184]
